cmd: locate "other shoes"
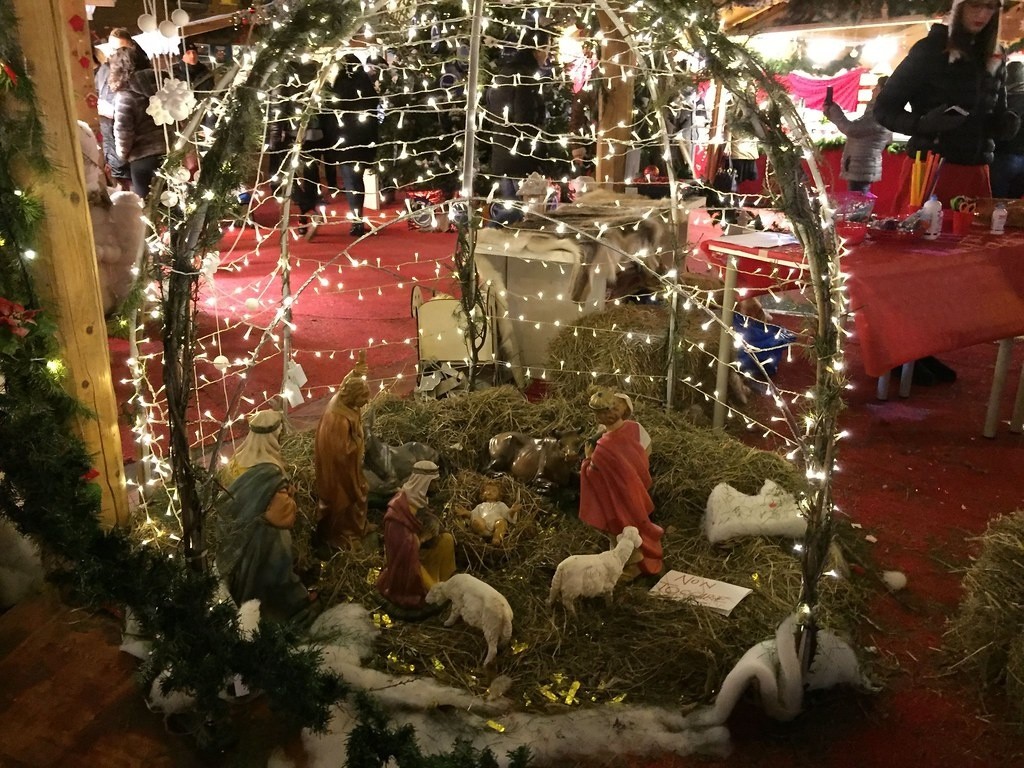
[350,218,364,237]
[893,352,956,386]
[298,220,308,235]
[305,211,317,229]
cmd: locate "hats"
[1007,61,1024,84]
[178,42,197,59]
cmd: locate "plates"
[867,214,928,243]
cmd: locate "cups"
[952,211,974,236]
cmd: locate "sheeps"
[548,526,643,618]
[425,573,514,664]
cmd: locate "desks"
[467,188,707,385]
[703,217,1024,441]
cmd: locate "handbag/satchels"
[716,153,737,193]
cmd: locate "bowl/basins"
[836,221,866,246]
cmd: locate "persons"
[578,390,664,575]
[315,362,376,555]
[455,481,522,546]
[380,461,457,613]
[207,410,320,634]
[96,0,1024,386]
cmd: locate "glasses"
[966,1,999,15]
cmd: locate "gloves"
[917,104,967,138]
[1002,110,1020,141]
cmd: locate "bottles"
[990,204,1008,235]
[921,195,942,240]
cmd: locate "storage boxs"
[733,314,803,373]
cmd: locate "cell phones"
[944,106,970,117]
[827,87,833,103]
